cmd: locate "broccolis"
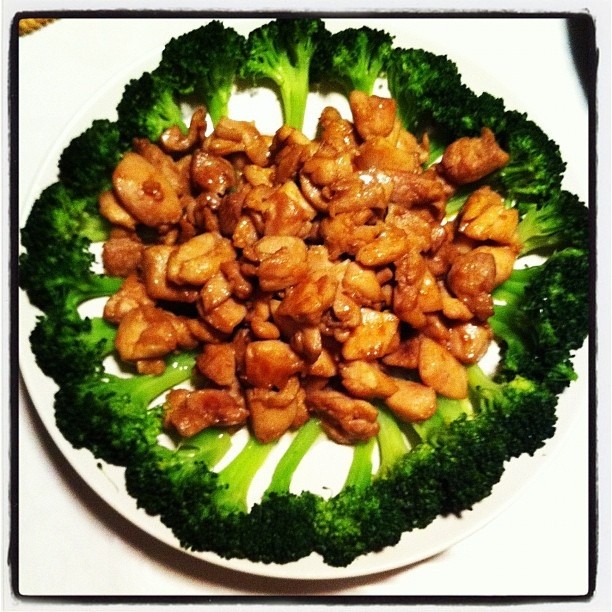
[17,16,591,567]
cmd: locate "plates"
[17,14,576,583]
